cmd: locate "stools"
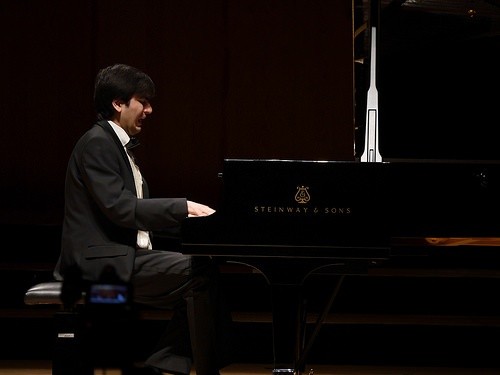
[25,281,135,375]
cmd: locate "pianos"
[181,159,500,375]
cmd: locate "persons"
[51,63,228,375]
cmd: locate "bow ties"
[126,138,142,151]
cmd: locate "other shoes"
[146,365,181,375]
